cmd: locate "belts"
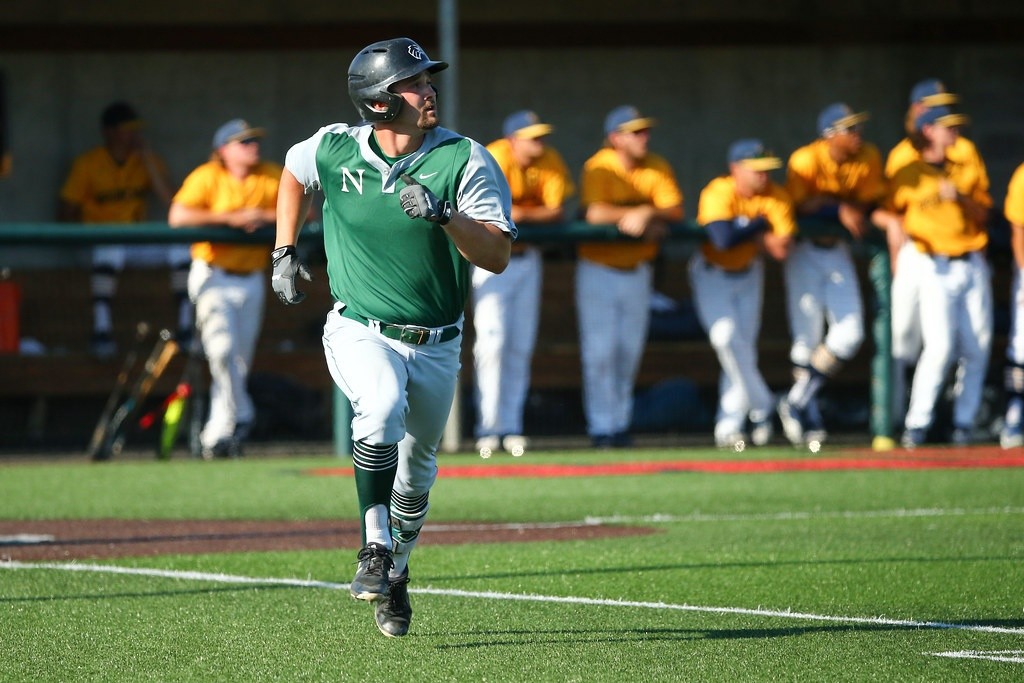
[339,305,460,345]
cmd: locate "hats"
[603,106,656,137]
[211,120,266,148]
[502,109,551,141]
[728,138,784,174]
[815,101,867,135]
[910,78,958,107]
[914,106,968,130]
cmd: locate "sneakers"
[374,564,413,638]
[349,542,396,601]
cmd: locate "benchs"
[8,259,873,456]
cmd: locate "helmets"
[345,36,449,124]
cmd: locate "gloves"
[399,174,452,226]
[268,245,316,306]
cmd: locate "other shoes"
[953,428,995,443]
[592,432,631,447]
[777,396,805,442]
[474,434,527,453]
[716,433,746,447]
[200,437,230,459]
[998,426,1024,450]
[750,407,774,444]
[902,427,926,446]
[806,421,827,446]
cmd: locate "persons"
[871,78,995,450]
[270,37,518,636]
[1000,160,1024,450]
[780,101,889,451]
[578,103,687,447]
[466,110,576,459]
[168,118,315,460]
[51,100,179,360]
[690,137,800,446]
